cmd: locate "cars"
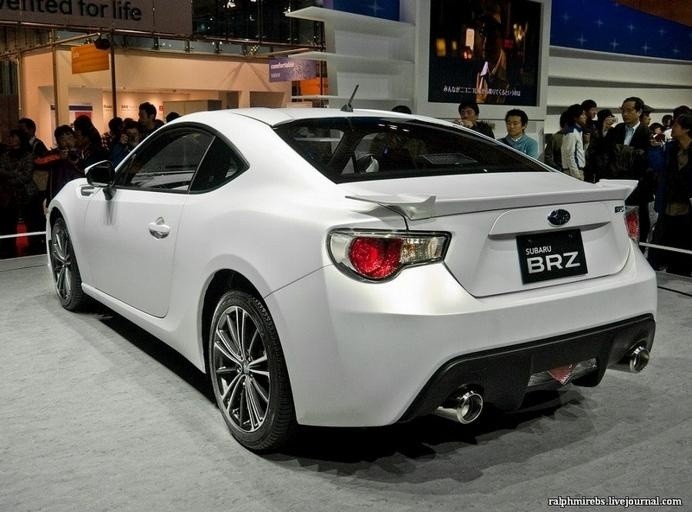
[40,80,664,455]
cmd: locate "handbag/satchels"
[32,170,49,192]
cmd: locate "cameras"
[60,144,78,162]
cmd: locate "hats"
[643,105,654,112]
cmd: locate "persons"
[546,97,692,276]
[0,102,183,259]
[454,100,494,138]
[471,4,519,104]
[370,105,425,171]
[496,109,538,159]
